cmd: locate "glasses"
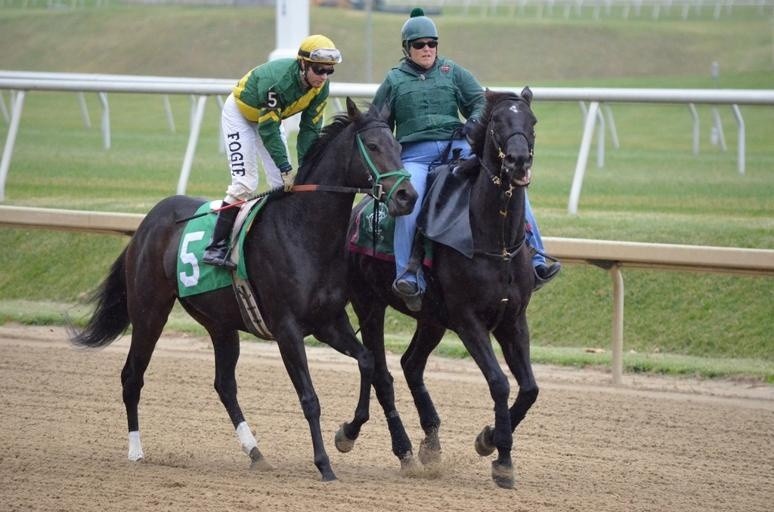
[410,40,437,49]
[305,63,334,75]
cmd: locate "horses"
[60,97,426,481]
[348,87,540,488]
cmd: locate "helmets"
[296,35,343,65]
[401,15,438,46]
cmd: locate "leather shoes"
[531,262,560,290]
[396,278,423,312]
[203,244,237,271]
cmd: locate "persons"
[368,6,562,312]
[201,34,342,271]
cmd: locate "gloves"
[461,118,487,145]
[280,168,298,193]
